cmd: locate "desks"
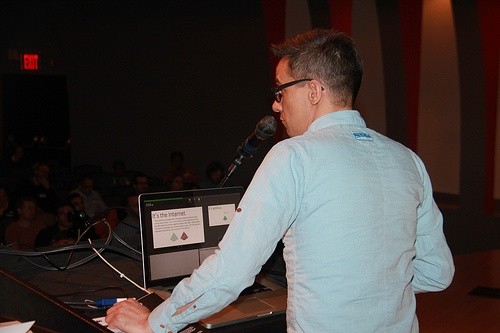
[0,235,286,333]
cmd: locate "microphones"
[227,116,277,172]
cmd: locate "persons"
[0,133,244,251]
[106,26,455,333]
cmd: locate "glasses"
[22,205,36,211]
[271,79,324,103]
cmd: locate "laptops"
[138,186,288,329]
